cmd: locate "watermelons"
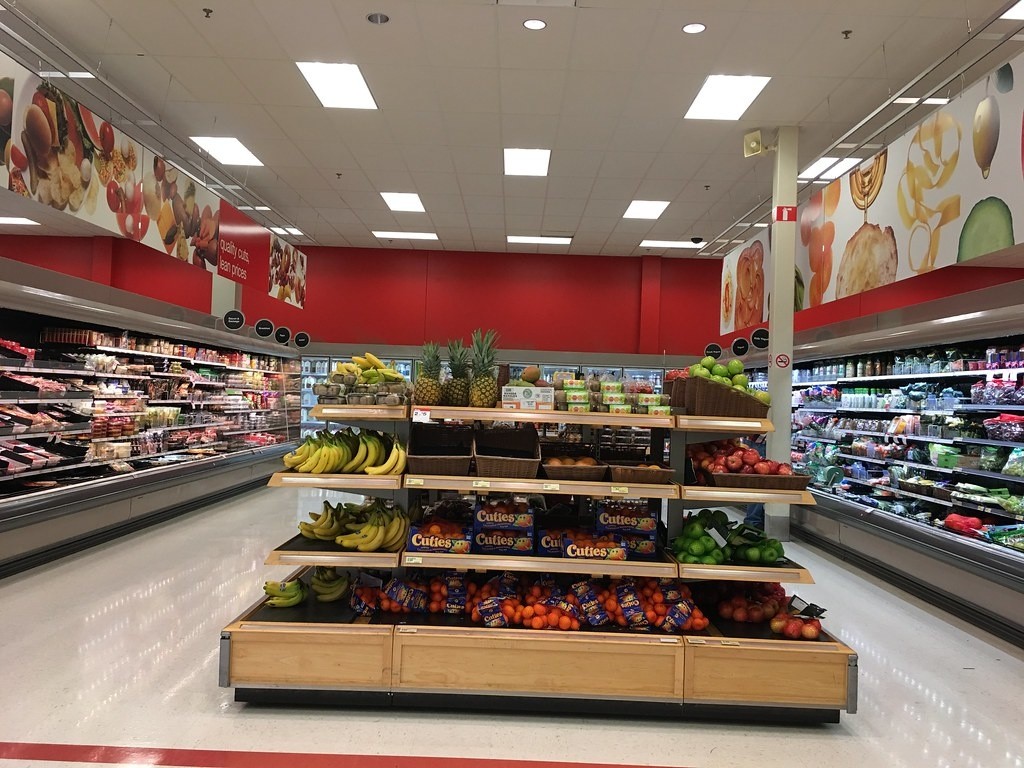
[76,102,104,151]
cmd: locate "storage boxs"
[473,500,534,531]
[598,531,658,558]
[561,532,629,561]
[502,386,554,410]
[937,453,980,469]
[405,506,473,554]
[536,521,597,556]
[595,500,658,534]
[473,528,534,556]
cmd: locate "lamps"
[882,44,960,105]
[104,72,173,127]
[827,97,888,149]
[235,177,280,213]
[33,16,114,80]
[200,157,250,190]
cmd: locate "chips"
[36,138,99,212]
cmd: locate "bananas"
[263,578,306,607]
[283,427,406,475]
[312,571,351,601]
[299,497,410,551]
[338,353,404,383]
[313,566,341,580]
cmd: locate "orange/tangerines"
[350,497,709,631]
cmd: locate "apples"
[155,158,178,202]
[687,437,795,483]
[719,582,821,638]
[99,121,114,152]
[667,357,770,404]
[673,507,784,565]
[0,89,12,127]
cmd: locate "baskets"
[663,376,771,419]
[685,458,812,491]
[406,422,675,484]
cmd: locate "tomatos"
[107,181,120,211]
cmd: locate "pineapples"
[445,341,470,406]
[414,339,442,405]
[470,327,498,407]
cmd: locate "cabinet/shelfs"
[0,256,302,580]
[716,281,1024,650]
[219,404,859,725]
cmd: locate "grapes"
[1002,462,1024,477]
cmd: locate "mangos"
[506,366,549,388]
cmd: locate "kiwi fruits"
[553,380,647,414]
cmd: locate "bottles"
[841,388,890,408]
[845,359,892,378]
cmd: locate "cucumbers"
[957,197,1014,264]
